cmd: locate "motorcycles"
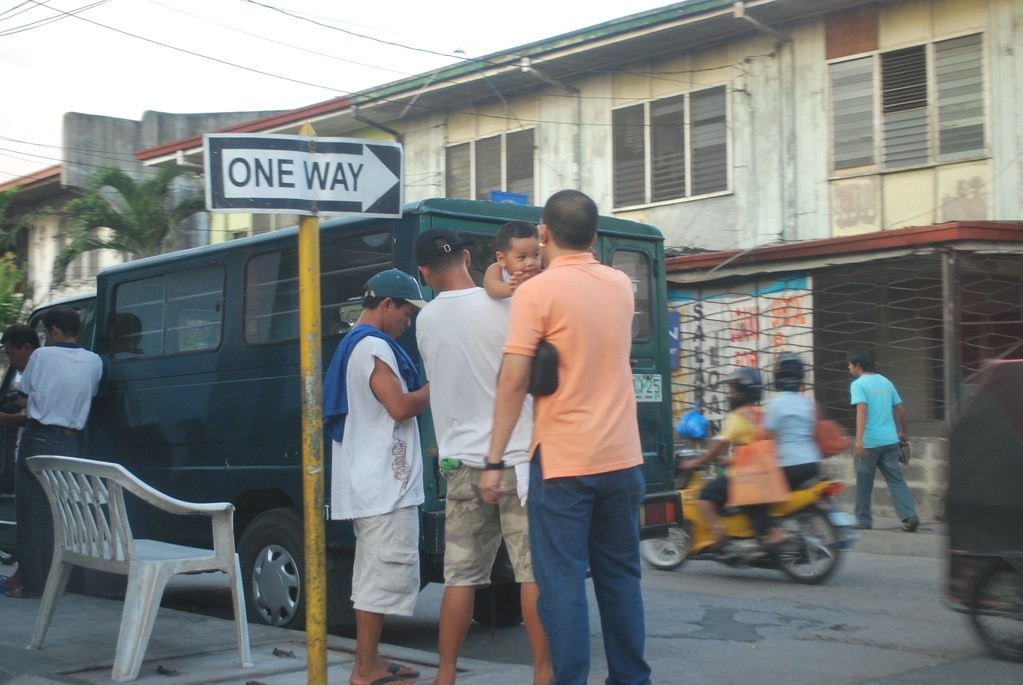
[638,447,842,585]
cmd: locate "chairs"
[26,454,253,682]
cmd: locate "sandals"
[7,587,28,597]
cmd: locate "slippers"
[370,675,405,685]
[388,662,419,679]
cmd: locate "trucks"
[0,196,685,632]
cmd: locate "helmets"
[727,367,762,390]
[774,353,803,378]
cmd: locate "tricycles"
[935,355,1022,666]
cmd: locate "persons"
[483,221,597,299]
[678,367,764,543]
[747,354,820,545]
[115,311,145,358]
[331,269,429,685]
[847,347,920,531]
[480,190,652,685]
[416,228,553,685]
[0,323,40,586]
[6,305,103,598]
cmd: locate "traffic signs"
[203,133,404,220]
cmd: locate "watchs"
[481,456,505,470]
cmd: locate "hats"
[415,227,473,286]
[365,268,428,308]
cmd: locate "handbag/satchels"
[495,341,557,395]
[723,414,787,508]
[815,403,848,458]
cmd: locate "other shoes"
[856,522,871,530]
[902,517,918,531]
